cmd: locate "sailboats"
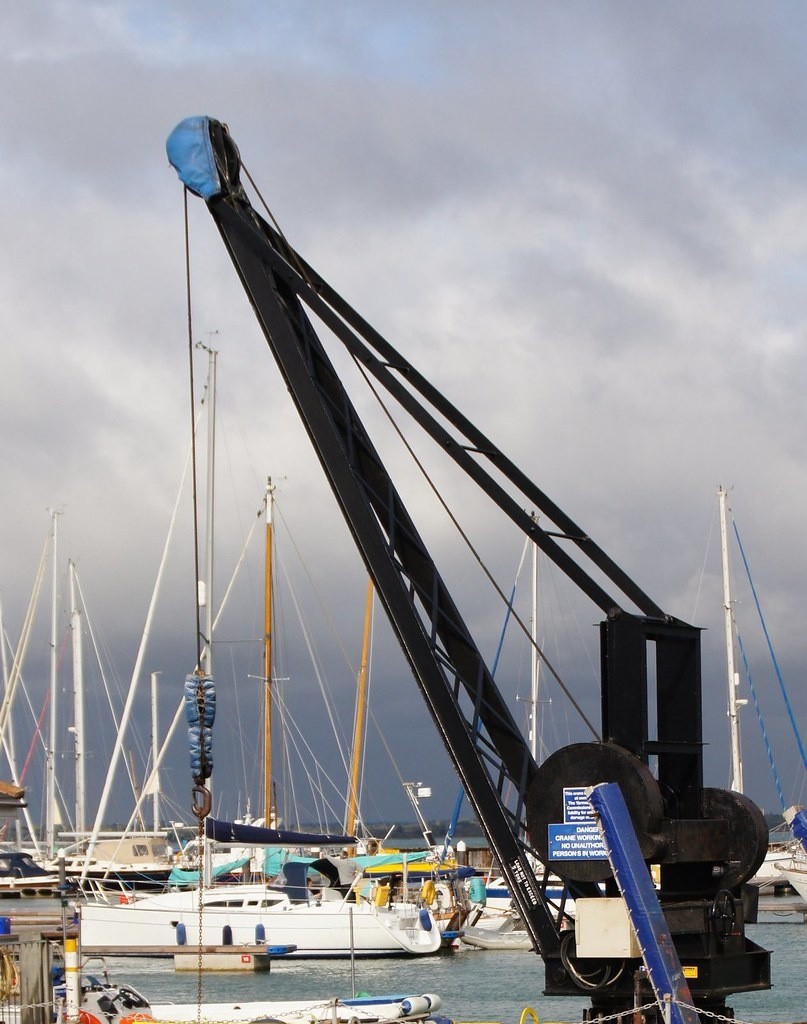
[2,339,805,1024]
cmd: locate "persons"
[305,878,321,907]
[325,850,361,899]
